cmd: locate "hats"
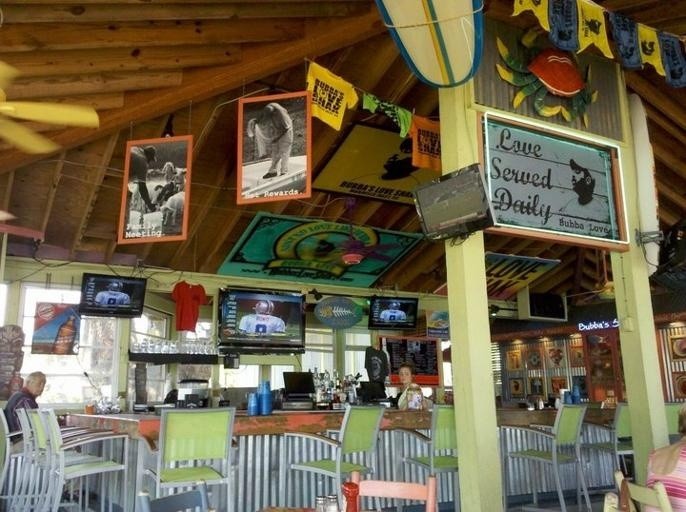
[144,146,157,162]
[570,157,594,177]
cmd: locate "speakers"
[530,293,564,316]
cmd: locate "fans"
[0,58,98,155]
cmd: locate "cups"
[537,385,581,410]
[129,340,216,354]
[84,398,121,415]
[246,380,271,416]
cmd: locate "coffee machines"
[173,378,209,410]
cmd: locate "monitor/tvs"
[659,217,686,276]
[413,164,494,244]
[78,273,147,315]
[219,289,306,347]
[368,297,418,332]
[360,381,386,399]
[283,372,316,394]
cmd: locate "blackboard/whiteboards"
[377,335,443,384]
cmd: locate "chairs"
[0,406,130,512]
[280,401,385,507]
[576,398,634,512]
[142,403,236,512]
[351,469,437,512]
[138,480,209,512]
[501,402,593,512]
[394,394,460,512]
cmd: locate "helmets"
[109,282,123,292]
[255,301,274,314]
[388,302,401,308]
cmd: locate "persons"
[397,362,423,410]
[380,302,406,320]
[123,145,186,239]
[239,299,285,333]
[4,372,47,443]
[255,102,294,178]
[646,403,686,512]
[561,157,610,222]
[94,281,131,305]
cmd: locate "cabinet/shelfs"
[583,329,628,402]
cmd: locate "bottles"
[51,315,76,354]
[309,365,357,411]
[314,480,360,511]
[406,383,424,411]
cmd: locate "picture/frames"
[672,371,686,398]
[668,335,686,362]
[507,344,584,398]
